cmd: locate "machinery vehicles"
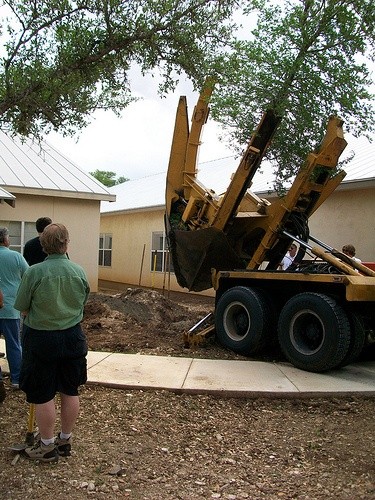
[164,75,375,372]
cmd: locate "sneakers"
[21,440,59,463]
[53,433,73,456]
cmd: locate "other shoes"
[12,384,20,391]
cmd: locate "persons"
[22,216,69,266]
[279,244,299,271]
[0,226,29,390]
[12,223,90,464]
[336,243,362,272]
